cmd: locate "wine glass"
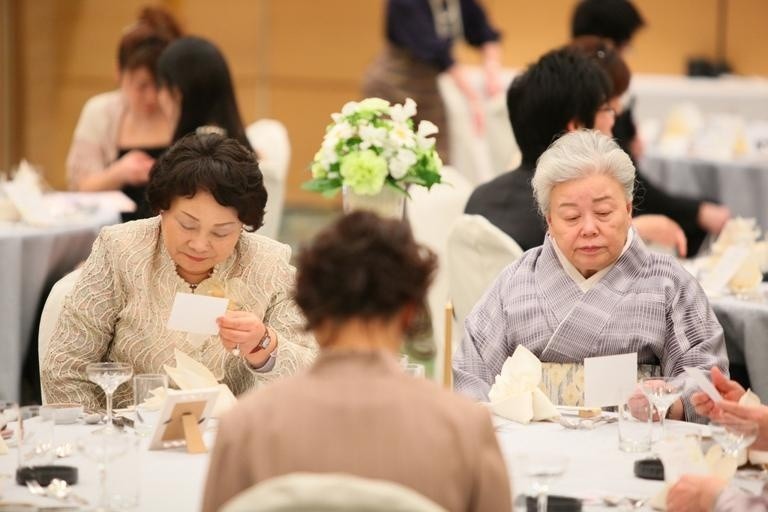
[640,377,687,426]
[85,359,134,450]
[708,420,761,460]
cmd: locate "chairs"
[222,116,294,247]
[444,213,529,331]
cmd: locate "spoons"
[86,412,103,426]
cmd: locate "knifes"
[111,410,134,431]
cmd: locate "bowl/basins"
[39,402,83,426]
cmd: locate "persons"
[367,1,503,165]
[64,8,184,225]
[201,209,512,511]
[666,474,766,510]
[690,366,767,451]
[151,35,255,156]
[449,132,731,428]
[463,0,731,263]
[40,130,319,415]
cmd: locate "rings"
[231,343,242,357]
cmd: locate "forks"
[561,414,620,433]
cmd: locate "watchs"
[249,326,272,356]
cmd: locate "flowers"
[306,91,448,207]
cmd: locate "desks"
[485,69,765,226]
[0,174,139,406]
[681,244,768,395]
[0,394,766,511]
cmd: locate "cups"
[398,353,424,378]
[100,456,144,512]
[18,405,57,467]
[134,374,167,426]
[618,380,653,455]
